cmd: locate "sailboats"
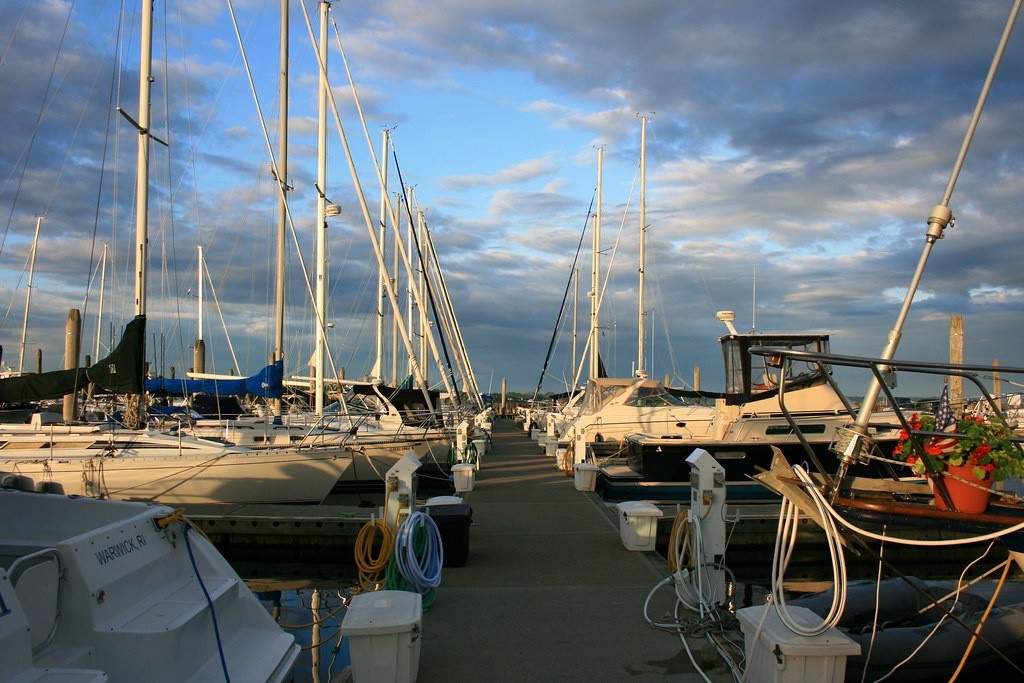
[522,1,1024,683]
[1,0,498,683]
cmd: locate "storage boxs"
[341,589,423,683]
[422,495,474,569]
[736,607,862,683]
[537,434,547,446]
[478,431,492,442]
[615,501,664,552]
[572,463,601,492]
[544,440,558,456]
[451,463,476,492]
[531,429,541,441]
[555,448,573,469]
[472,440,486,456]
